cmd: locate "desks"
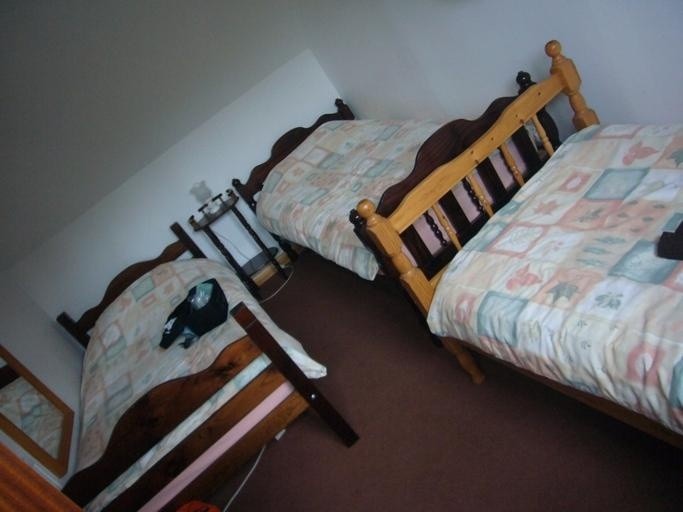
[188,188,294,303]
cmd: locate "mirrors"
[0,345,75,478]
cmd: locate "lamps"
[189,181,220,214]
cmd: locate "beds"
[232,70,562,350]
[56,222,360,512]
[356,39,683,451]
